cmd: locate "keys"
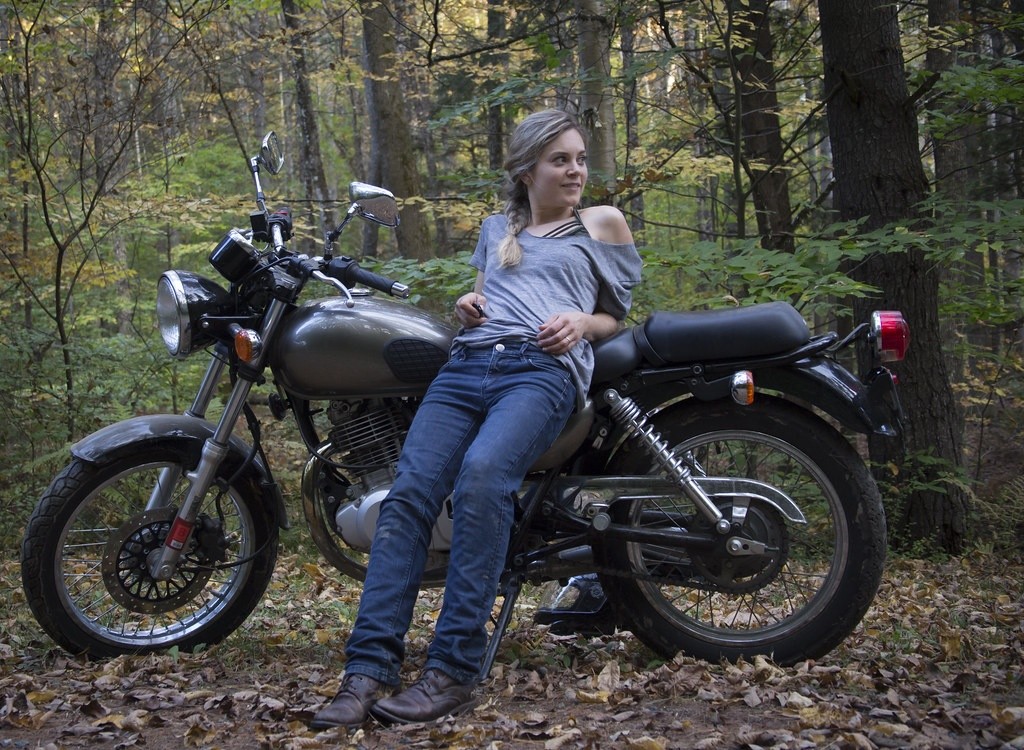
[471,301,485,318]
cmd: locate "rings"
[565,336,571,342]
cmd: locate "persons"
[311,110,643,730]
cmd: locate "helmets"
[533,573,629,639]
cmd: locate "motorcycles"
[21,131,908,669]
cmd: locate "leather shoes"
[368,668,480,730]
[310,673,403,730]
